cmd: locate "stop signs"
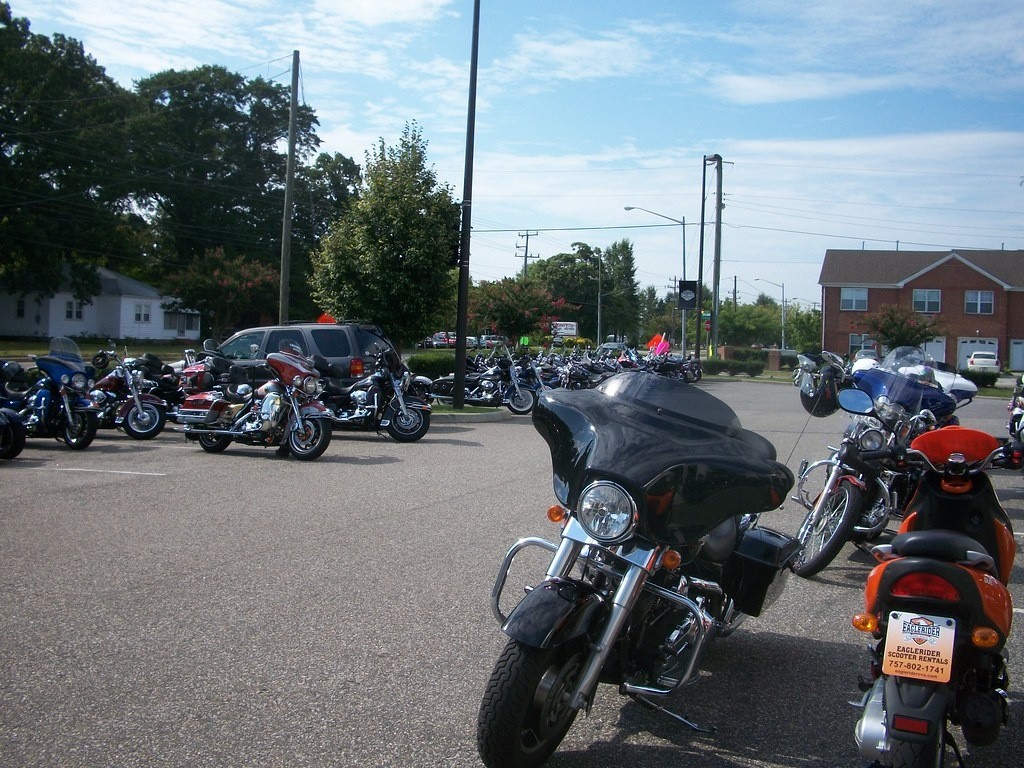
[705,320,711,331]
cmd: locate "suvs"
[164,323,392,392]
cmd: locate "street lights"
[753,278,785,349]
[624,205,687,358]
[792,297,816,309]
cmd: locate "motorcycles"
[476,373,804,768]
[0,334,431,464]
[794,344,1023,766]
[432,336,702,415]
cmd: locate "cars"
[965,351,1000,377]
[751,345,761,350]
[553,338,563,347]
[434,332,470,348]
[417,337,450,348]
[467,337,488,348]
[601,342,627,354]
[852,348,881,365]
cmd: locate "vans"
[482,334,511,348]
[606,335,628,344]
